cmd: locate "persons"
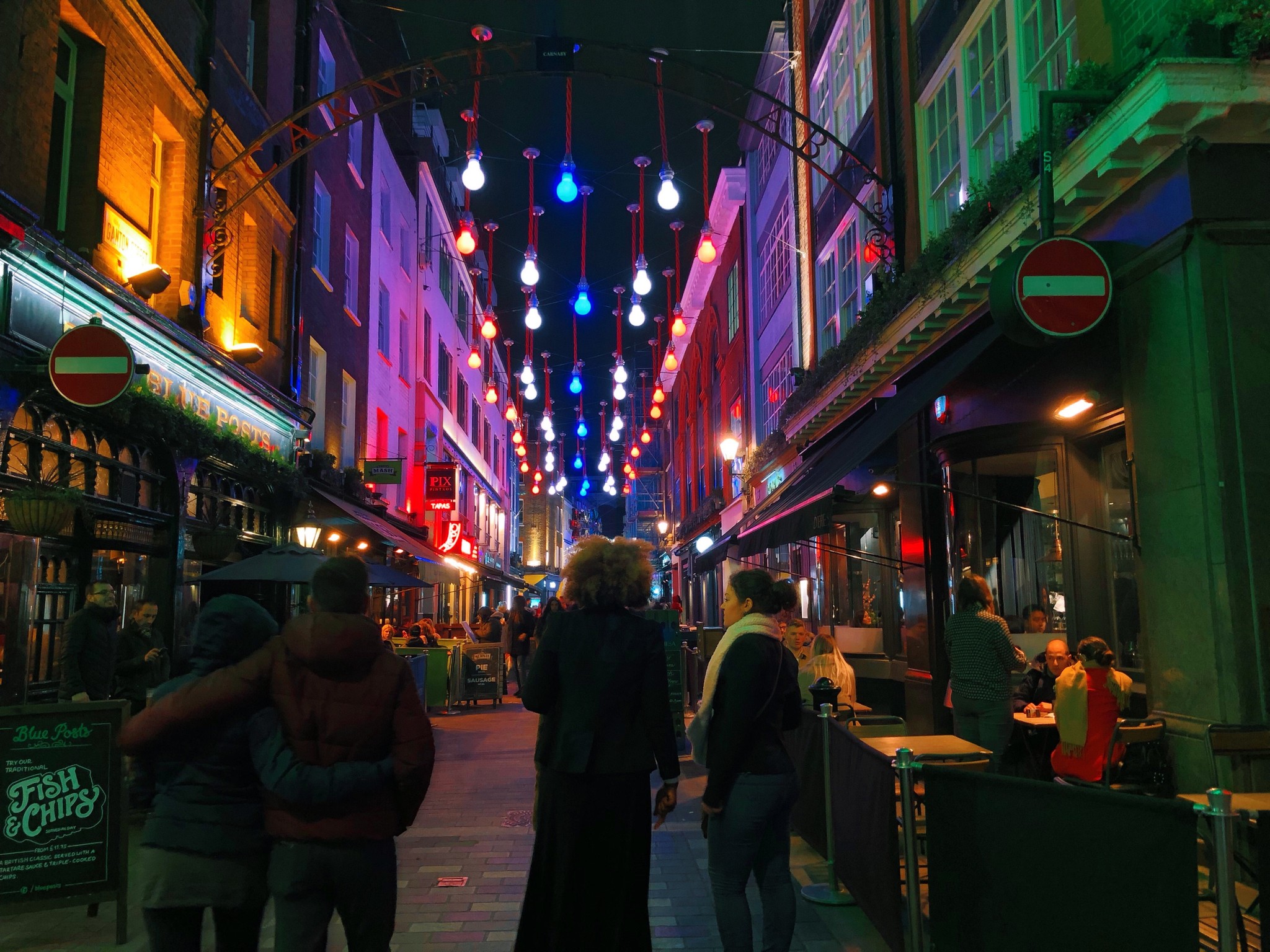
[525,597,544,618]
[797,633,861,726]
[58,579,121,704]
[473,606,504,643]
[1051,637,1132,787]
[116,598,170,716]
[785,618,811,668]
[779,620,787,642]
[944,574,1028,775]
[498,602,509,621]
[505,595,535,698]
[515,537,681,952]
[659,595,684,621]
[1013,640,1077,713]
[131,593,396,952]
[381,618,441,647]
[119,552,436,952]
[686,568,803,952]
[851,610,871,628]
[1004,604,1046,633]
[534,596,566,640]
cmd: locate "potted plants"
[343,466,364,494]
[2,448,98,540]
[183,500,247,561]
[313,449,338,486]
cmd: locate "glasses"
[90,589,117,596]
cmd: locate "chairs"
[832,702,855,728]
[845,715,991,882]
[1204,722,1270,912]
[1063,717,1168,794]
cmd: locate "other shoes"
[514,691,521,696]
[516,694,522,698]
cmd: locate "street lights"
[657,517,669,601]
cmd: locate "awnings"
[309,484,543,601]
[694,321,1131,580]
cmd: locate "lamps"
[291,498,324,551]
[372,492,384,500]
[230,343,265,365]
[304,430,313,442]
[719,432,745,480]
[121,264,172,302]
[1055,394,1095,419]
[656,517,673,546]
[408,512,417,521]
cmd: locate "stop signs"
[48,324,135,408]
[1012,235,1116,338]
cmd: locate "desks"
[860,735,993,854]
[1176,791,1270,951]
[1011,713,1125,780]
[837,701,872,713]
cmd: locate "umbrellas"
[185,540,433,618]
